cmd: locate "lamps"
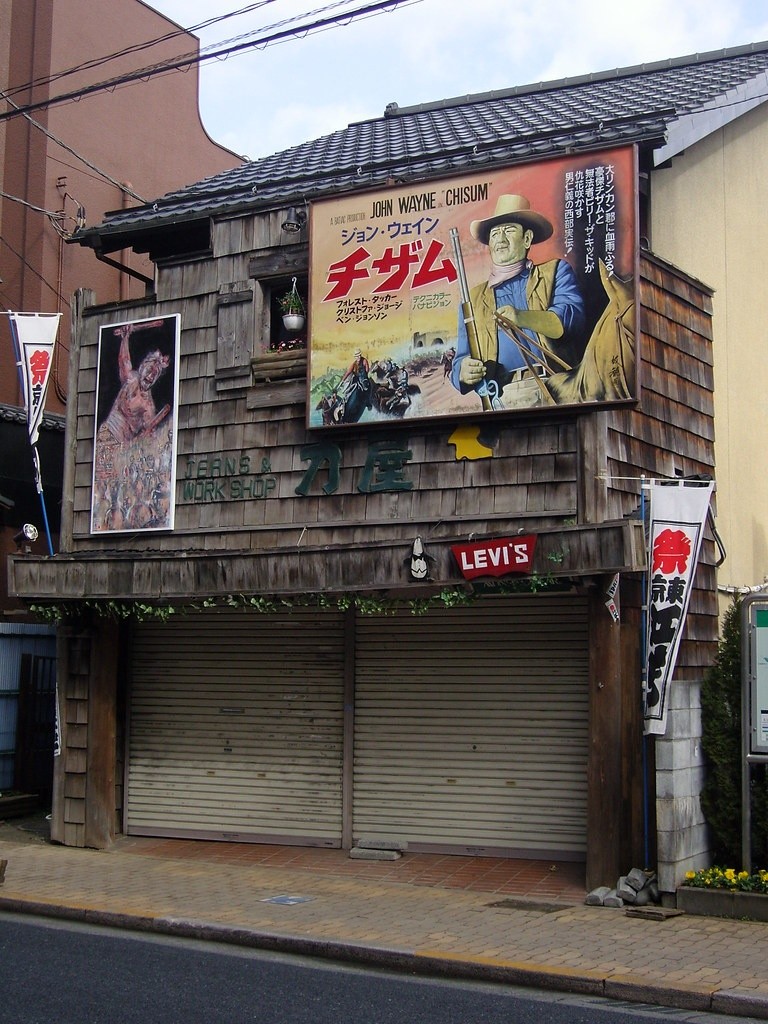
[12,524,39,551]
[281,208,306,234]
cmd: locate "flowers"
[276,291,306,314]
[682,865,768,894]
[261,339,305,354]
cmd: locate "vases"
[282,314,304,331]
